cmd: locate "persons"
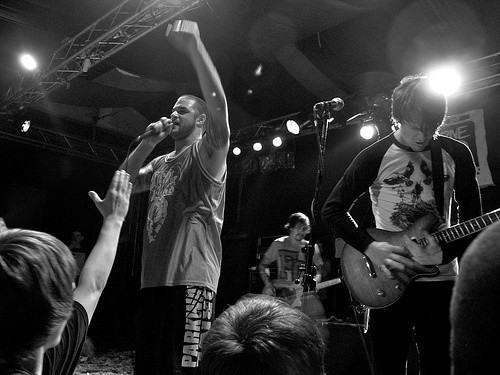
[0,170,132,375]
[320,75,483,375]
[67,228,91,288]
[258,213,322,312]
[449,218,500,375]
[118,20,230,375]
[199,293,327,375]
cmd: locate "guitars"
[262,278,341,302]
[340,208,500,309]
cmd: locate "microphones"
[133,119,174,143]
[313,97,344,111]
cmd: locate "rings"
[378,265,387,271]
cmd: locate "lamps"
[286,112,310,135]
